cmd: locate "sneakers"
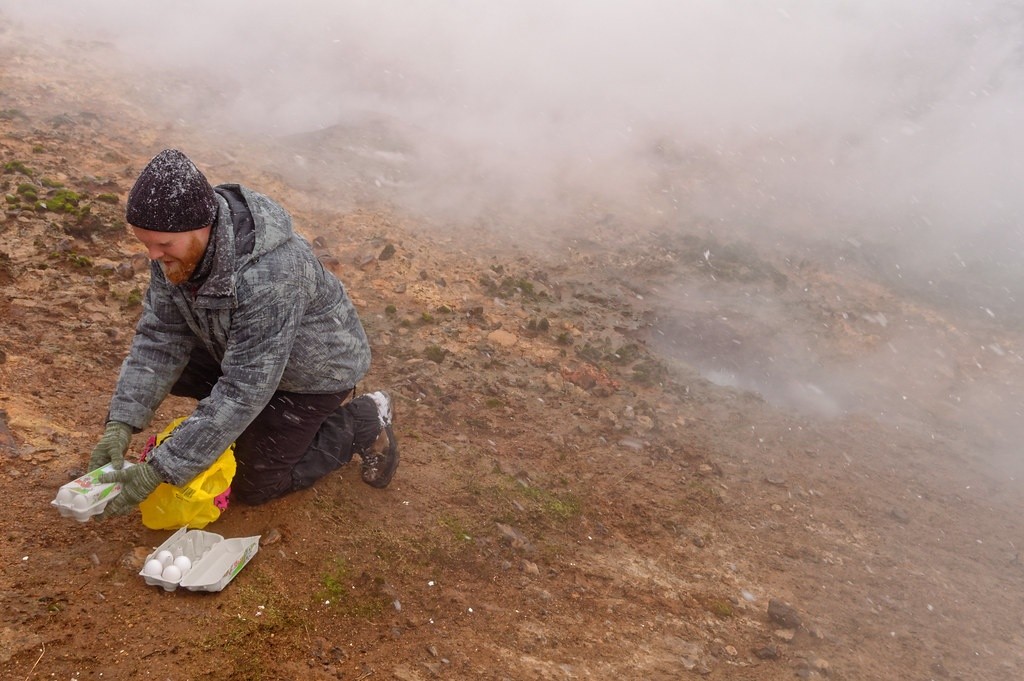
[353,390,400,488]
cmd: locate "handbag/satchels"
[140,415,238,530]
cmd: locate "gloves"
[88,420,132,473]
[94,461,163,522]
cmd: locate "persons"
[89,148,402,522]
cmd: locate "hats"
[126,149,218,233]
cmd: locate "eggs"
[144,550,191,582]
[55,489,87,510]
